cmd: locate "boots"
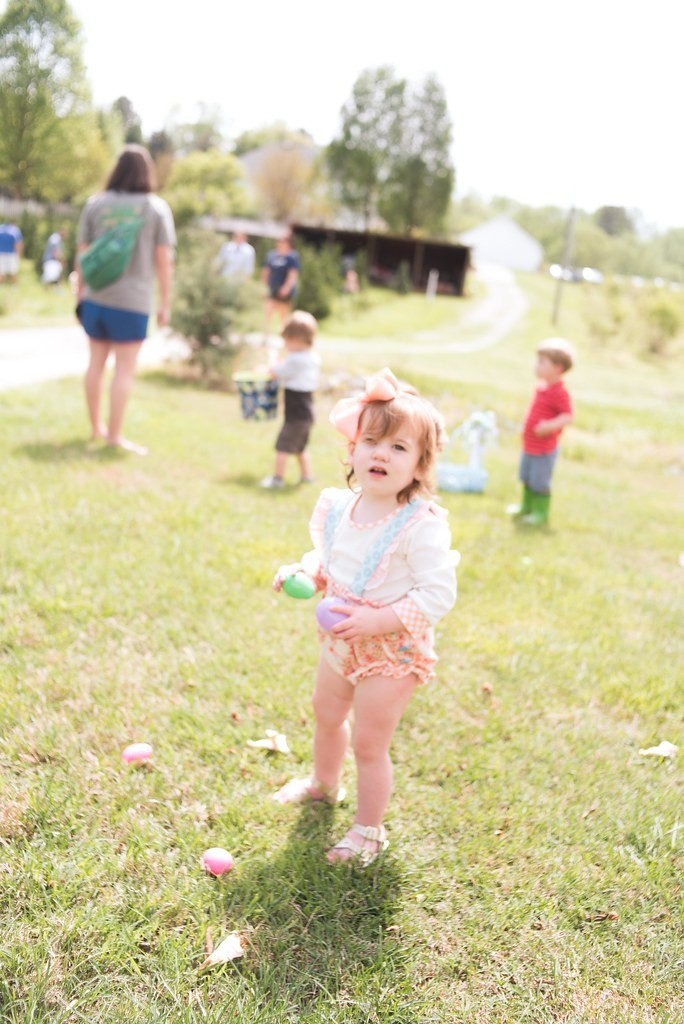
[511,486,551,527]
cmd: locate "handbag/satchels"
[78,217,144,290]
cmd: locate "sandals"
[323,825,390,869]
[271,768,347,807]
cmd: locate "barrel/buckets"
[232,373,280,421]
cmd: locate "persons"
[270,390,460,871]
[0,214,71,288]
[73,144,177,457]
[257,309,318,491]
[508,345,575,530]
[209,226,301,320]
